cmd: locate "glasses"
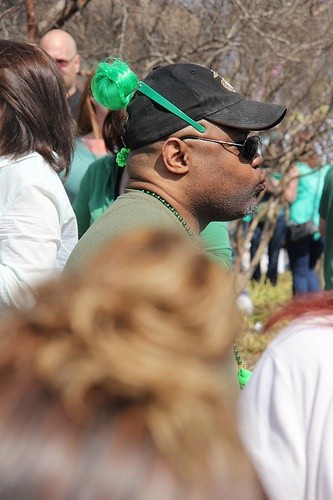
[178,134,261,163]
[55,54,77,67]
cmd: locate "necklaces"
[123,186,245,369]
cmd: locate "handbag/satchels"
[286,217,318,243]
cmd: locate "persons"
[37,29,96,138]
[58,62,291,282]
[0,221,273,498]
[0,40,78,313]
[57,67,115,212]
[227,130,333,307]
[71,103,234,266]
[234,289,333,500]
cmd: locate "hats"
[122,63,288,155]
